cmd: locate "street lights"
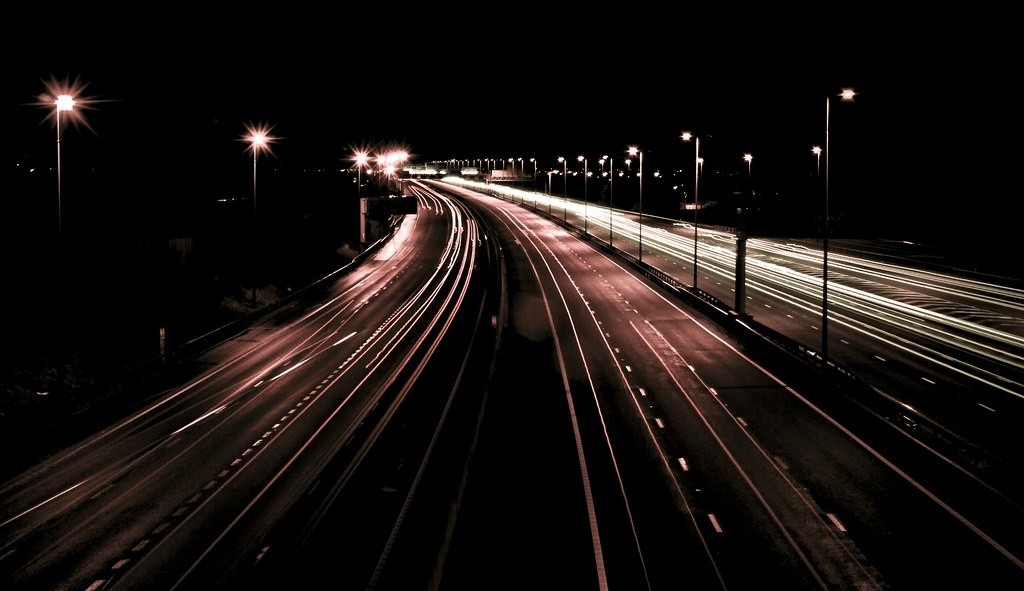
[628,146,642,265]
[557,157,567,224]
[249,131,266,209]
[698,157,704,178]
[602,155,613,248]
[356,153,366,195]
[811,145,823,242]
[578,156,587,235]
[743,153,753,180]
[377,151,407,195]
[680,130,699,289]
[820,88,858,359]
[444,157,538,194]
[54,93,75,226]
